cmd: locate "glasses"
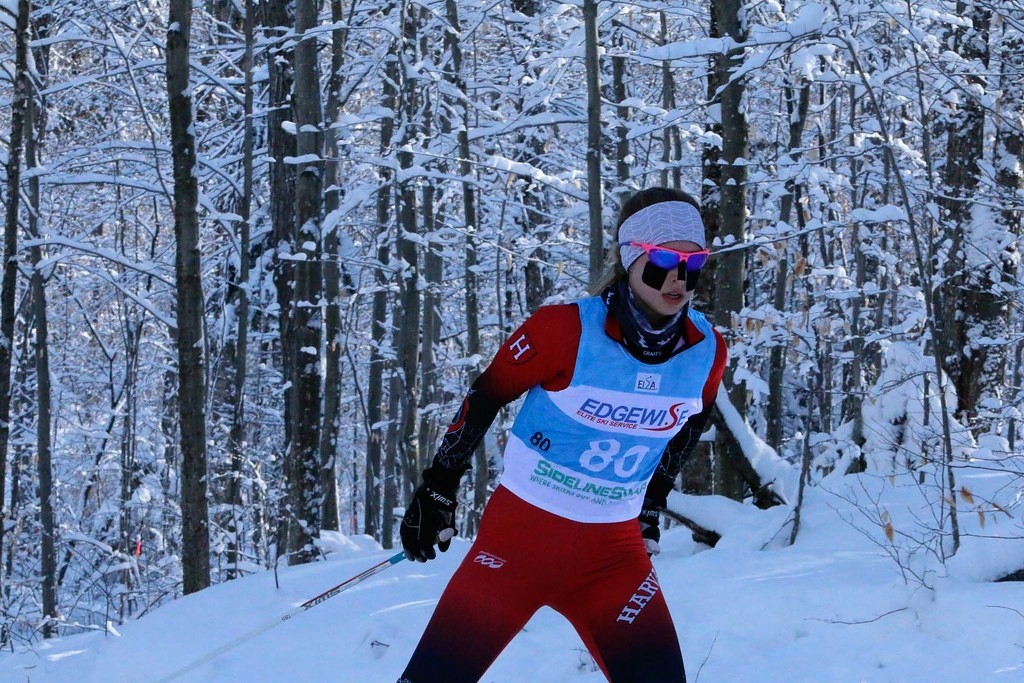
[617,242,709,272]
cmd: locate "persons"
[394,187,728,683]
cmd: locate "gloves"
[637,471,674,560]
[399,453,473,563]
